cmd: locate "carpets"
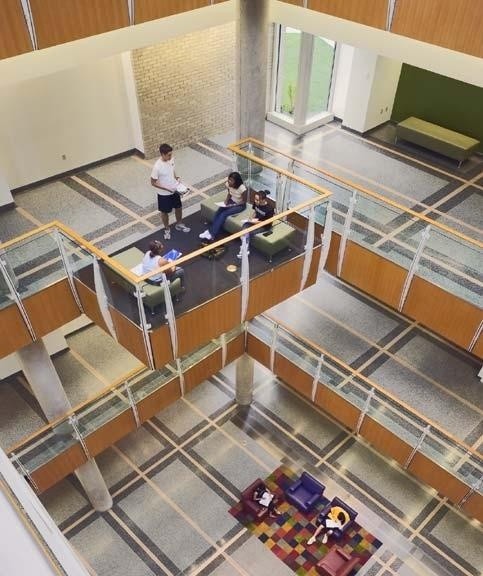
[228,464,383,576]
[74,208,321,332]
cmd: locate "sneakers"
[199,229,212,240]
[175,224,191,233]
[164,228,170,240]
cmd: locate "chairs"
[240,478,279,522]
[284,472,325,513]
[316,496,359,540]
[315,544,360,576]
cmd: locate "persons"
[306,506,350,545]
[150,144,191,240]
[236,190,274,260]
[252,482,281,519]
[198,172,248,241]
[142,240,185,288]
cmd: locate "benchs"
[200,190,296,262]
[394,116,479,168]
[102,247,185,316]
